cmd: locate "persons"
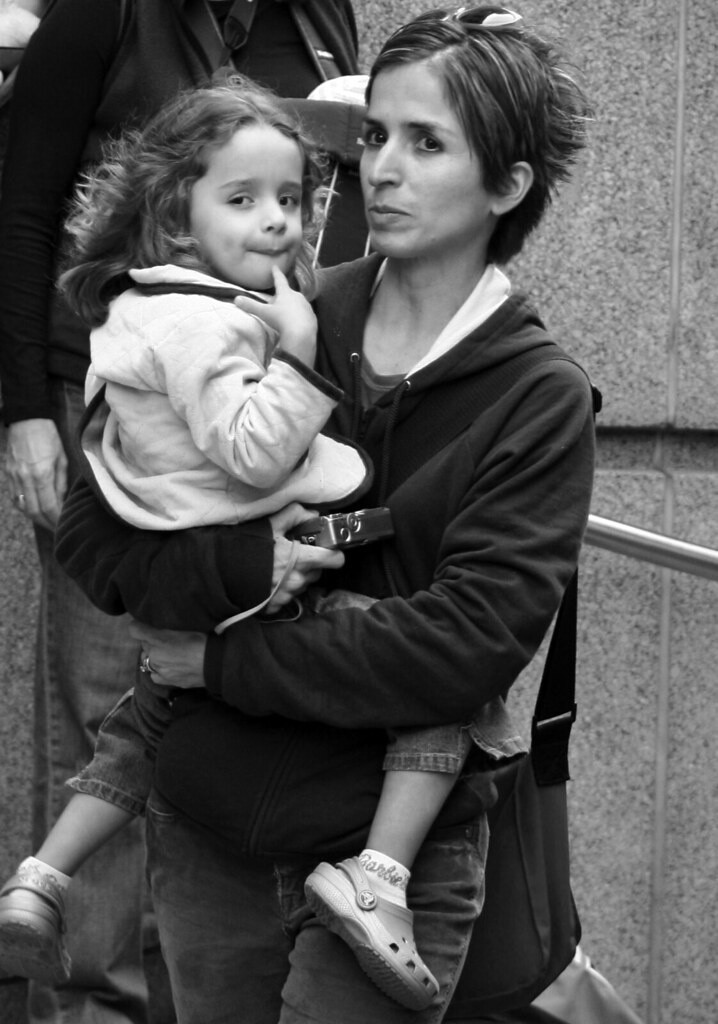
[0,76,476,1010]
[0,1,370,1024]
[149,15,596,1024]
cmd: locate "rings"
[14,495,25,502]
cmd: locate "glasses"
[402,5,525,34]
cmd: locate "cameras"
[283,507,393,550]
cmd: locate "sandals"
[0,871,70,987]
[304,857,439,1011]
